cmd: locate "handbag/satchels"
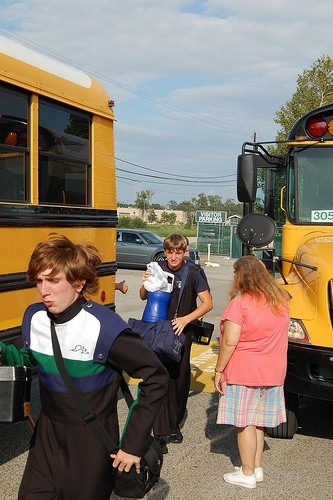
[108,435,164,500]
[127,318,186,367]
[189,321,214,345]
[142,262,175,293]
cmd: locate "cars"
[116,228,200,271]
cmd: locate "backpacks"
[186,264,211,342]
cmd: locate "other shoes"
[169,426,183,443]
[159,435,168,454]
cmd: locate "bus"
[0,24,119,367]
[236,102,333,439]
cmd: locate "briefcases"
[0,365,31,424]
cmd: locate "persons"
[139,233,213,443]
[215,255,290,489]
[0,232,170,500]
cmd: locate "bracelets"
[215,368,222,373]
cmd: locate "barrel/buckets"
[141,271,175,323]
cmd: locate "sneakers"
[233,466,264,482]
[222,468,257,488]
